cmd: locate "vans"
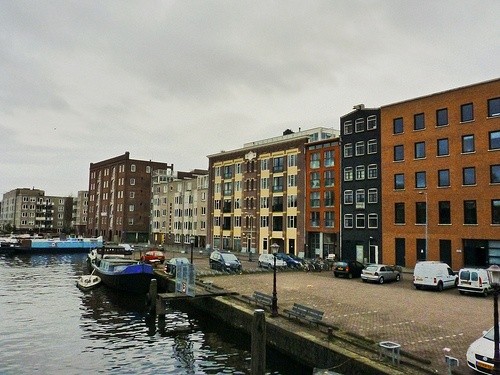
[456,267,495,297]
[413,261,459,292]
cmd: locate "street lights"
[189,234,196,264]
[269,243,281,317]
[486,264,500,375]
[418,191,428,259]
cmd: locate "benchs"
[247,290,275,313]
[282,302,324,329]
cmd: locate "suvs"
[210,250,243,272]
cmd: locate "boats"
[76,243,155,300]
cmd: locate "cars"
[118,243,134,254]
[258,252,304,271]
[333,260,363,279]
[466,325,494,375]
[142,251,165,264]
[164,257,194,277]
[360,262,401,284]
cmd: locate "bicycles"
[296,257,329,272]
